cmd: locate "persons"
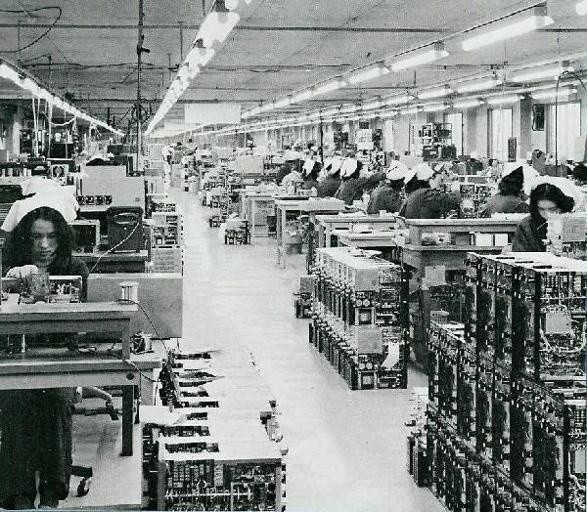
[0,199,88,510]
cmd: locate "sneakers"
[38,496,60,510]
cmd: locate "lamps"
[142,1,242,138]
[0,57,126,138]
[172,0,584,140]
